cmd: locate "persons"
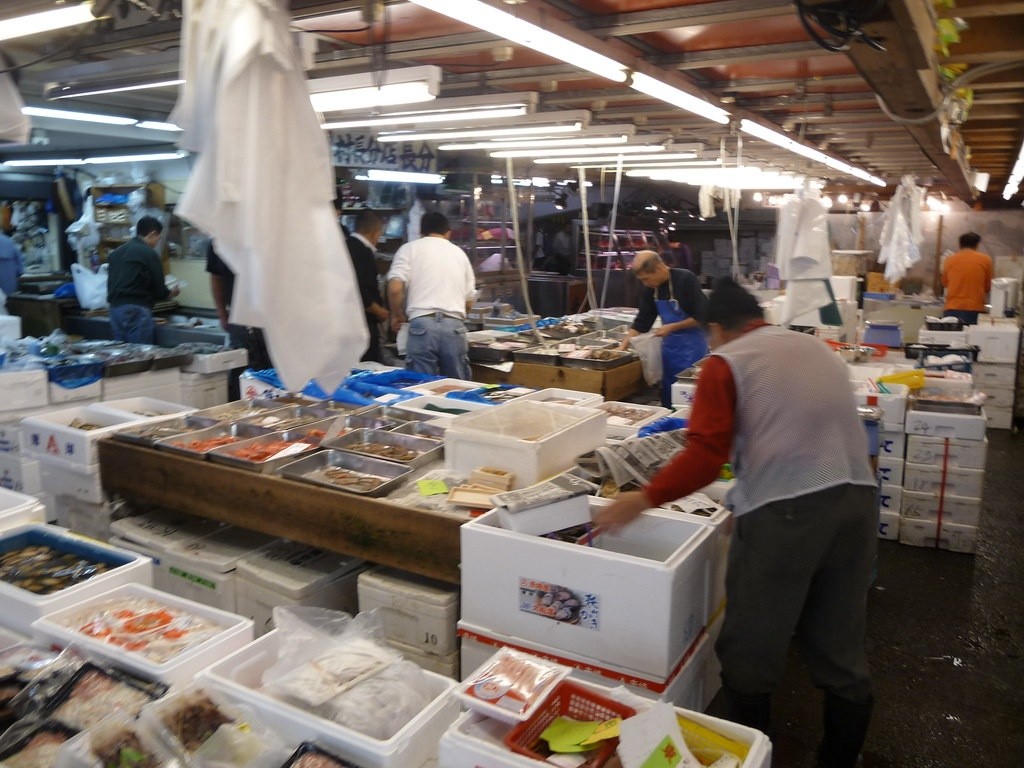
[385,212,478,383]
[206,239,274,402]
[615,250,709,409]
[939,232,992,325]
[0,234,24,295]
[666,232,692,270]
[345,208,389,364]
[590,276,892,768]
[551,222,572,261]
[107,216,179,344]
[534,222,545,266]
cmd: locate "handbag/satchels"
[73,263,112,310]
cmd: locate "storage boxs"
[0,249,1024,768]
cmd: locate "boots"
[721,676,776,737]
[818,690,875,768]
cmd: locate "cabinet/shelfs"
[338,169,677,311]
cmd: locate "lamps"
[853,192,862,209]
[860,192,878,210]
[0,0,890,191]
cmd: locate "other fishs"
[325,466,385,493]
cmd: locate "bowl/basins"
[837,345,875,364]
